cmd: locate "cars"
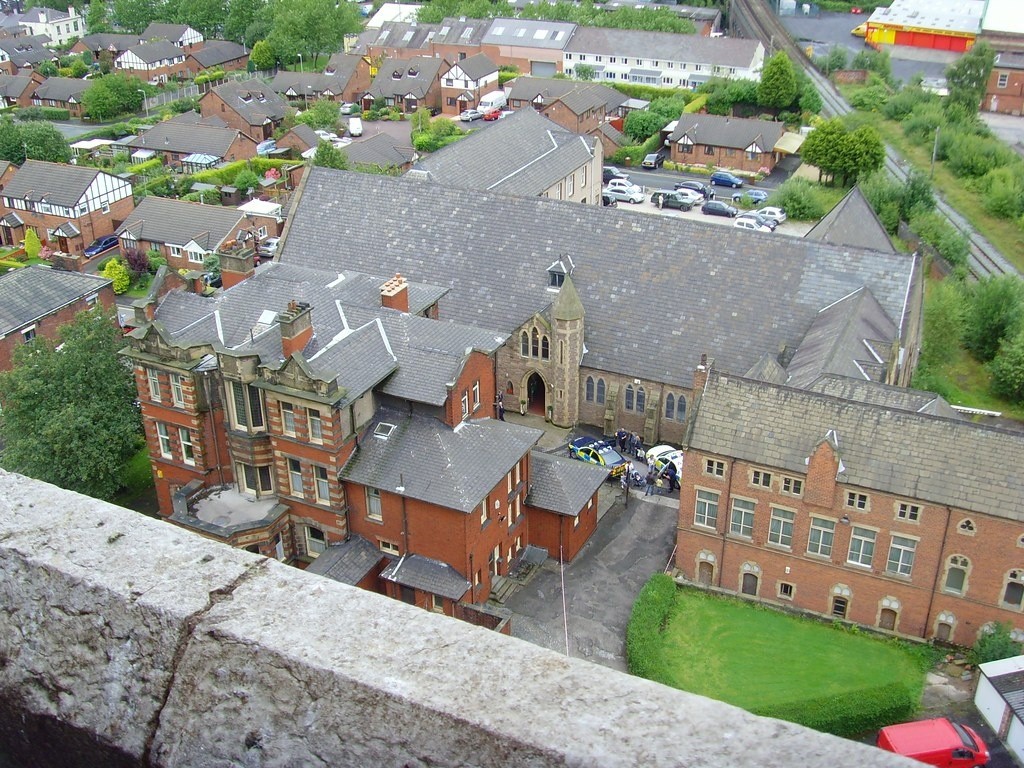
[426,105,441,116]
[645,444,684,490]
[732,205,787,233]
[256,236,282,257]
[731,189,768,205]
[83,234,119,259]
[641,153,666,169]
[204,272,223,288]
[459,108,503,122]
[338,103,361,115]
[568,436,635,481]
[701,199,738,218]
[709,171,744,189]
[601,166,716,210]
[252,256,262,267]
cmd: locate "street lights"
[297,54,304,72]
[137,89,149,117]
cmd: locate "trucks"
[347,117,363,137]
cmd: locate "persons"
[497,390,505,420]
[701,185,717,201]
[615,427,676,496]
[658,193,664,210]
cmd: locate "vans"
[875,717,993,768]
[476,90,507,114]
